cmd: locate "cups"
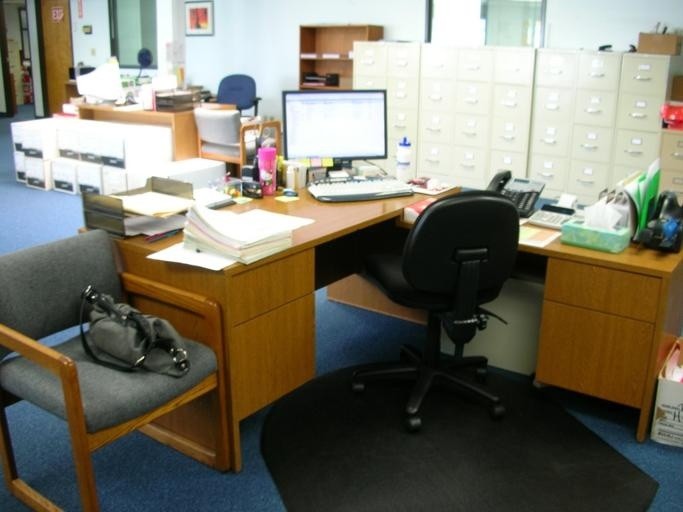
[258,149,274,196]
[283,167,299,191]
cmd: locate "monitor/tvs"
[282,90,387,169]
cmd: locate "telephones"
[486,168,546,218]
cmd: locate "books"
[181,202,293,265]
[300,51,353,86]
[616,159,663,244]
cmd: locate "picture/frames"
[181,2,217,40]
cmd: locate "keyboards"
[308,177,413,201]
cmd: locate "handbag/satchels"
[79,285,191,378]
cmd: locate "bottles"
[395,137,410,182]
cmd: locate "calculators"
[526,203,576,231]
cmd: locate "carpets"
[258,349,662,510]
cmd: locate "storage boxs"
[632,29,679,58]
[8,119,171,197]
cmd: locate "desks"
[76,187,462,473]
[407,189,682,441]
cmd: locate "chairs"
[347,188,524,437]
[208,72,263,121]
[192,108,282,178]
[1,227,231,511]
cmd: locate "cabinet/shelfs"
[526,44,671,203]
[346,36,418,187]
[658,130,682,209]
[416,39,537,191]
[297,23,382,93]
[75,97,237,171]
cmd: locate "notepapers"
[329,170,349,178]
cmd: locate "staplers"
[234,181,264,199]
